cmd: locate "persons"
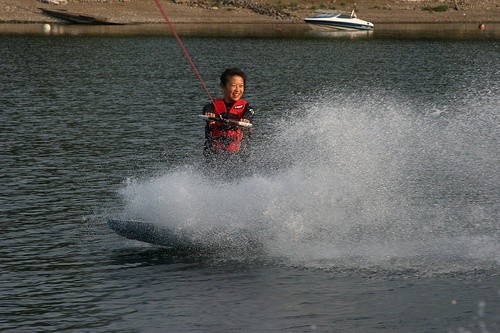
[203,68,255,180]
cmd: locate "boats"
[303,9,375,28]
[307,22,373,37]
